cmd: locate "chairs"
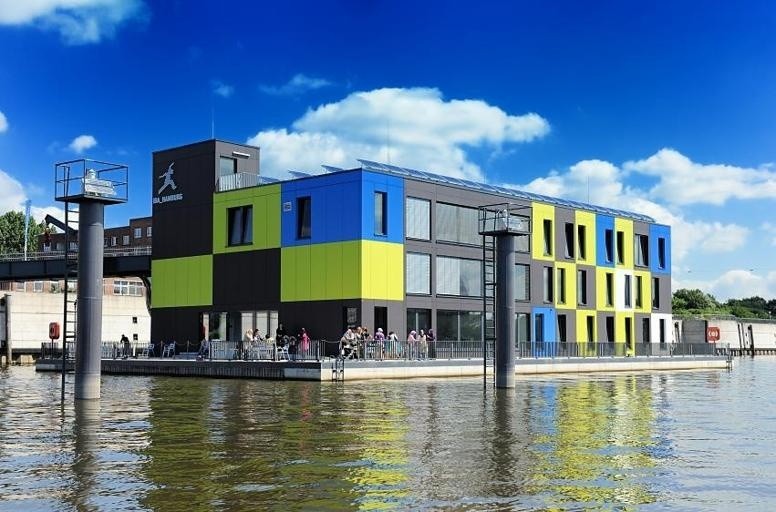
[142,339,385,361]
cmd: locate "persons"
[120,334,130,356]
[241,326,438,362]
[197,339,207,355]
